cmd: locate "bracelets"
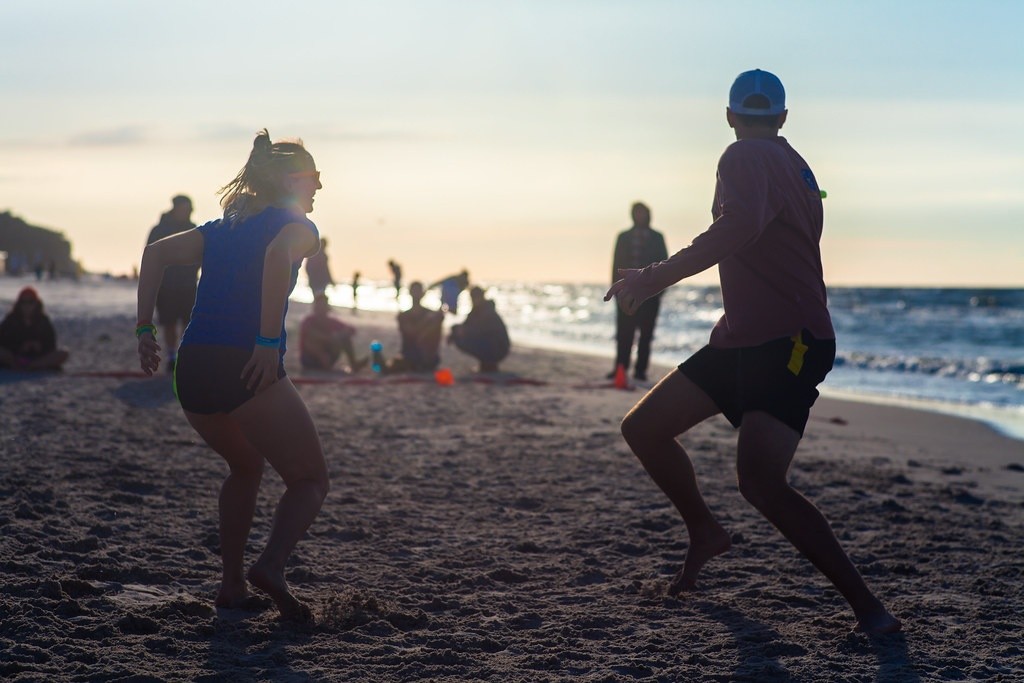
[136,321,157,341]
[256,335,282,351]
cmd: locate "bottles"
[371,340,383,373]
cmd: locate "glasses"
[287,171,322,183]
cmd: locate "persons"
[301,239,511,373]
[605,203,669,380]
[603,67,900,641]
[136,126,330,623]
[146,196,201,374]
[0,287,69,381]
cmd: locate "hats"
[19,286,38,302]
[728,69,786,116]
